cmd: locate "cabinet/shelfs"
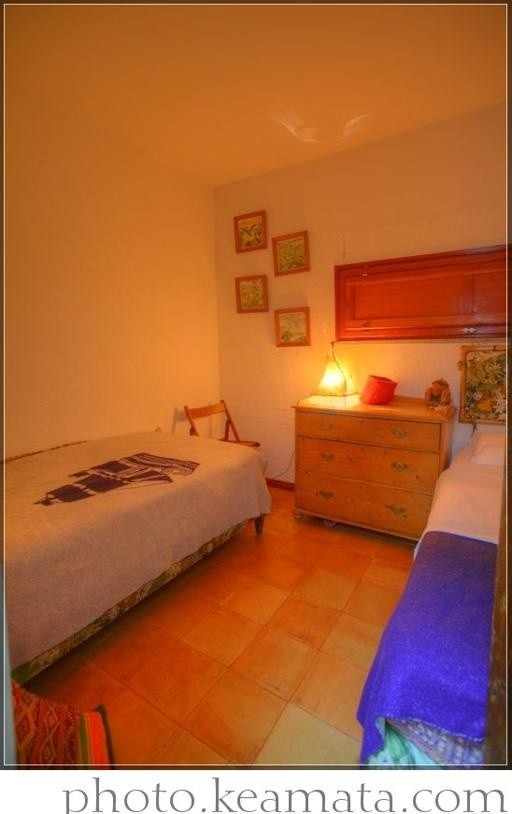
[290,395,458,543]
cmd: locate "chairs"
[183,400,260,448]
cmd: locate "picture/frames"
[458,345,511,426]
[234,209,312,347]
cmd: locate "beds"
[357,447,505,769]
[0,429,272,687]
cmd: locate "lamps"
[315,340,359,396]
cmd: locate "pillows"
[12,679,118,771]
[471,432,506,468]
